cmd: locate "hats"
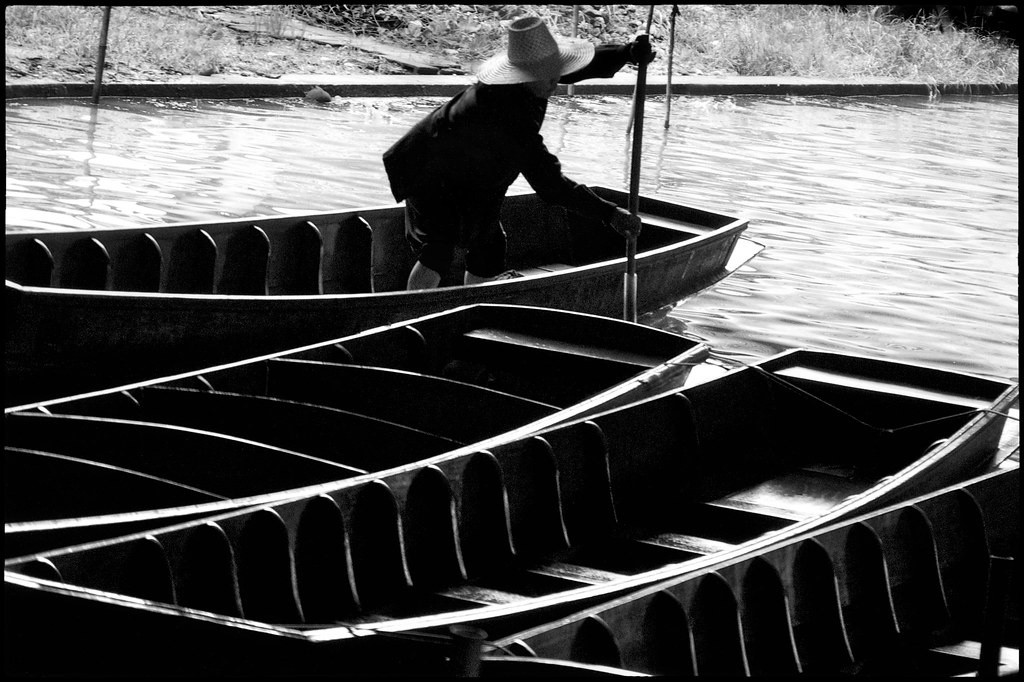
[476,17,594,85]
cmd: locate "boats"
[0,296,715,563]
[0,344,1019,682]
[0,180,753,372]
[365,461,1024,682]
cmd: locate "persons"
[384,19,657,290]
